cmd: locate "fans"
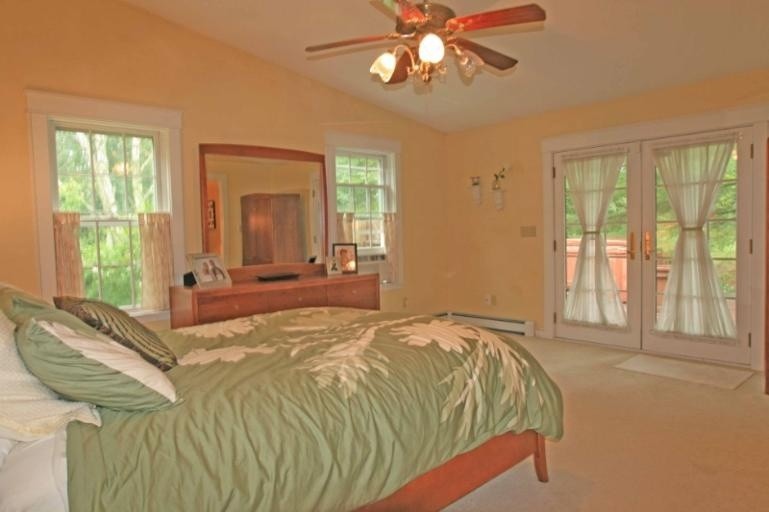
[303,1,548,86]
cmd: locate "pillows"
[0,282,186,455]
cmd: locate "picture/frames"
[332,243,358,274]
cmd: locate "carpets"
[612,353,757,391]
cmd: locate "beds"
[0,305,549,511]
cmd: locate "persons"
[340,249,350,268]
[200,259,225,284]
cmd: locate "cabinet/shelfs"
[240,193,304,266]
[167,272,381,330]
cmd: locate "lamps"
[368,32,484,82]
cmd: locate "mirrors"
[198,141,328,274]
